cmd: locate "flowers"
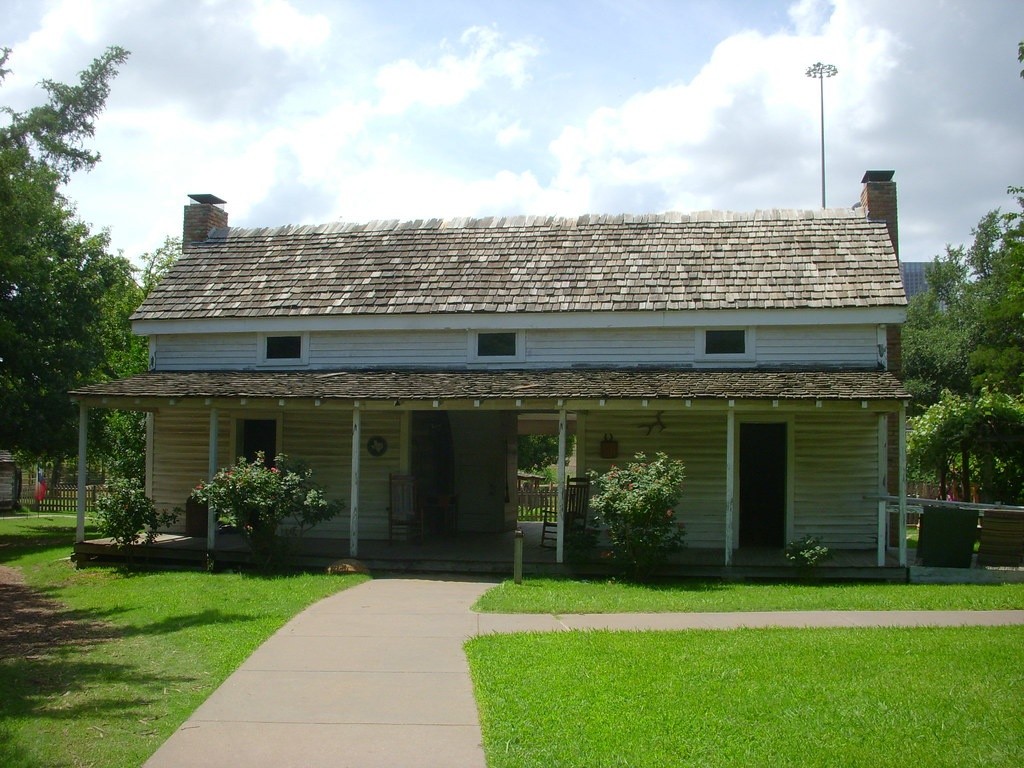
[665,524,688,554]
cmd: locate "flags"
[34,466,46,501]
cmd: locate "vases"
[666,552,681,566]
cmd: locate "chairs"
[540,475,590,550]
[388,473,425,543]
